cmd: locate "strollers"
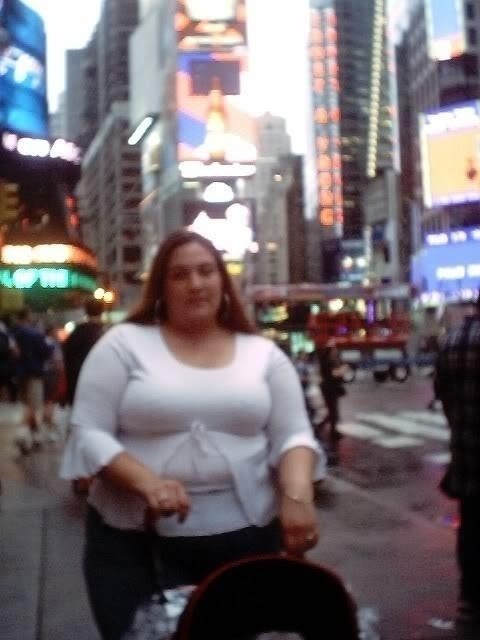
[124,506,362,640]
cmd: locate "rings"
[159,492,168,503]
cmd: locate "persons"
[1,299,106,455]
[295,337,343,451]
[434,291,480,640]
[59,230,329,639]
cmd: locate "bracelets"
[278,485,317,505]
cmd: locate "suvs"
[334,342,411,382]
